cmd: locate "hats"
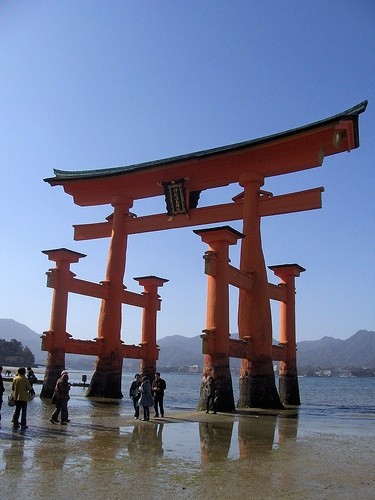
[61,370,68,375]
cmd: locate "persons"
[0,366,5,420]
[11,367,32,430]
[5,370,11,377]
[50,374,71,426]
[53,370,71,422]
[151,372,167,417]
[136,376,154,421]
[129,374,141,419]
[127,423,164,455]
[27,367,37,397]
[205,376,216,414]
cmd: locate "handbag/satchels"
[30,375,37,384]
[7,394,16,407]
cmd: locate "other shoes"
[54,419,60,422]
[11,420,21,424]
[140,417,149,421]
[61,422,67,425]
[134,417,138,420]
[13,425,20,428]
[21,426,28,429]
[50,419,55,425]
[66,419,71,423]
